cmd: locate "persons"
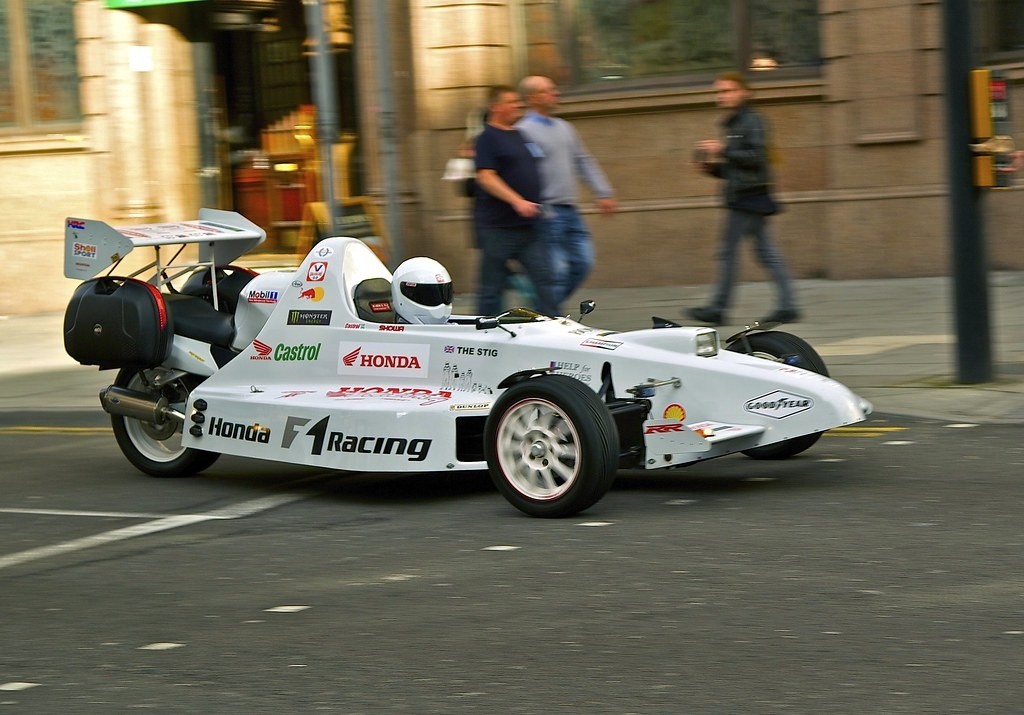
[688,73,799,324]
[458,75,619,317]
[391,257,461,326]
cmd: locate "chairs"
[353,278,397,325]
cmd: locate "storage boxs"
[64,276,173,371]
[181,263,259,314]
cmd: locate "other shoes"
[760,309,799,325]
[685,306,722,324]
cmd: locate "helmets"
[391,256,453,325]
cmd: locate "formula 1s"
[63,207,874,518]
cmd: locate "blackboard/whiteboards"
[311,197,390,260]
